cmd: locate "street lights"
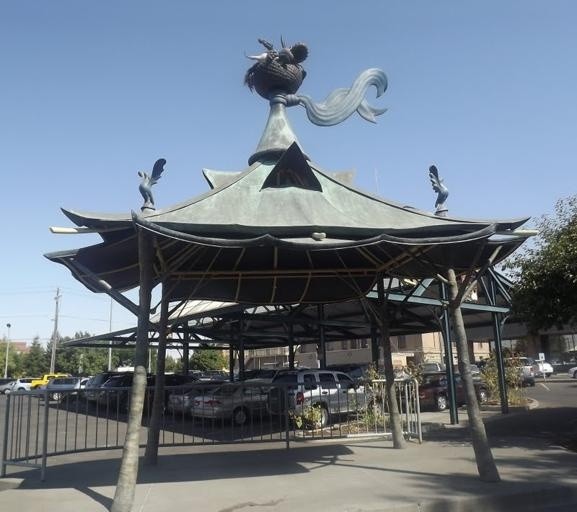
[4,322,14,377]
